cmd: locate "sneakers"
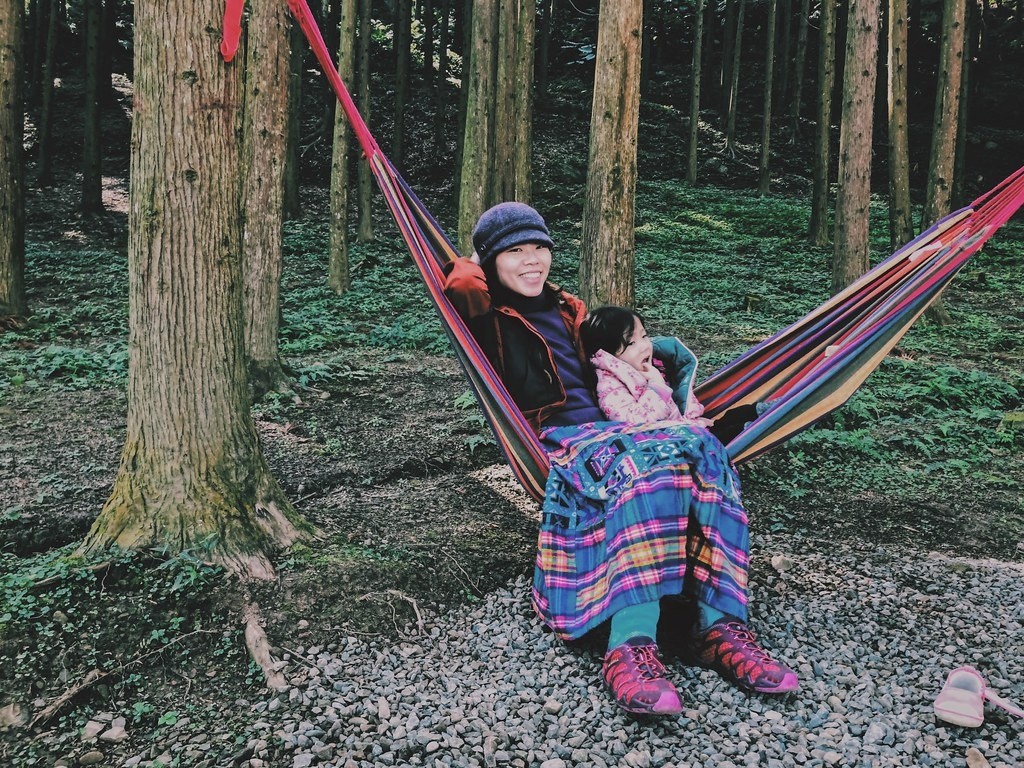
[680,619,797,696]
[603,641,683,716]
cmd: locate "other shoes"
[933,667,1024,728]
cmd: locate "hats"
[472,202,555,262]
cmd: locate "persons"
[443,200,799,718]
[579,306,783,448]
